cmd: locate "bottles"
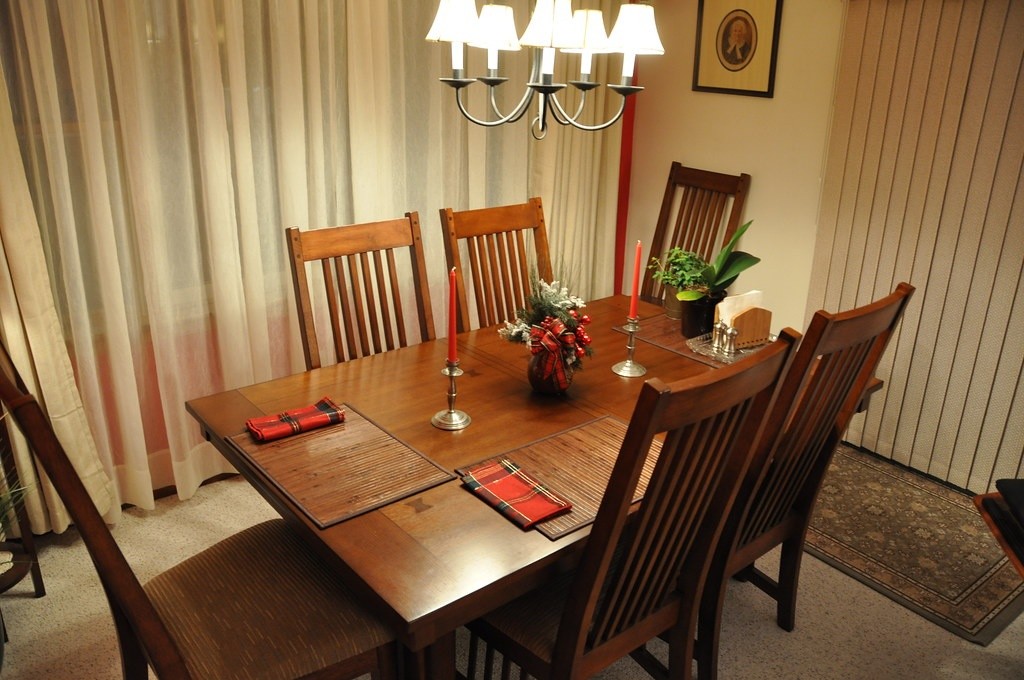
[711,318,727,349]
[724,326,739,354]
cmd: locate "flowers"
[499,282,597,350]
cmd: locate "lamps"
[425,0,665,140]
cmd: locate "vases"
[528,342,574,394]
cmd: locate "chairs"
[641,160,752,314]
[437,196,560,333]
[458,324,803,680]
[630,280,916,679]
[0,338,420,680]
[286,211,437,371]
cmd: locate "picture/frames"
[692,0,783,98]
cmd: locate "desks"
[184,296,885,679]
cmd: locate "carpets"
[802,443,1024,648]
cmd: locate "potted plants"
[650,221,757,334]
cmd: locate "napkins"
[245,396,346,444]
[460,455,572,532]
